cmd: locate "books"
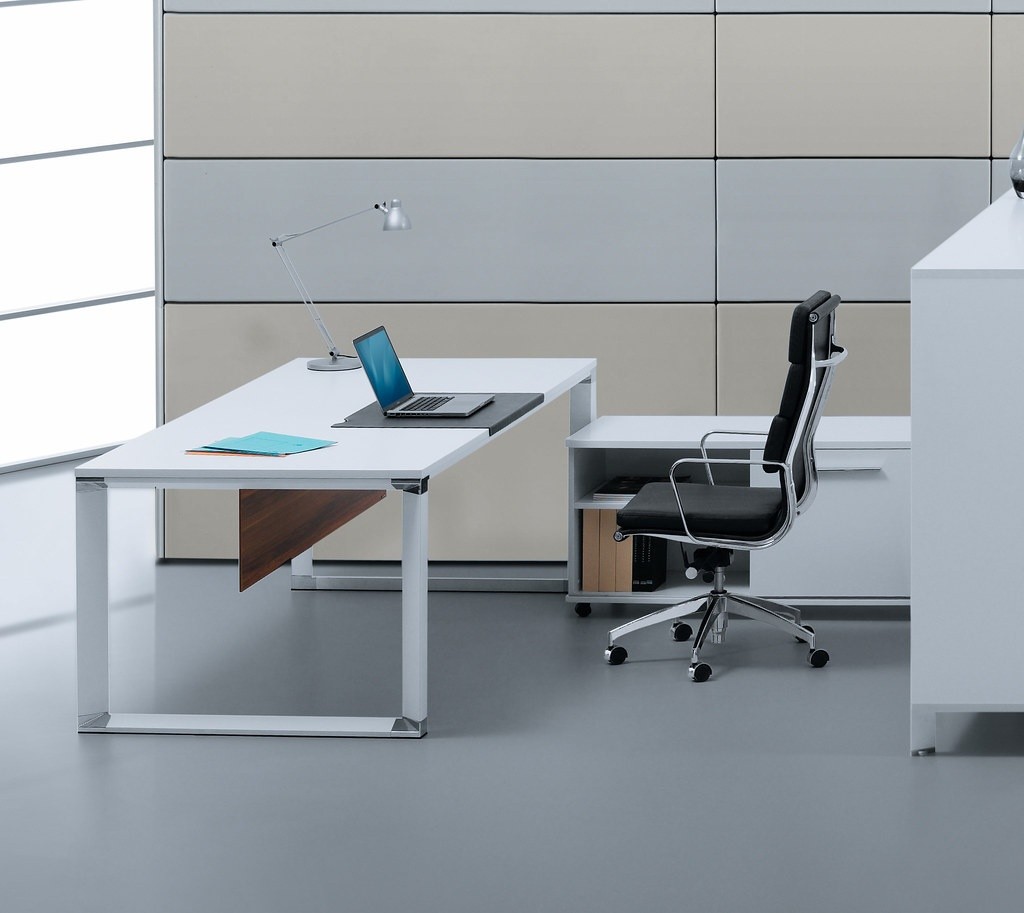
[593,475,691,501]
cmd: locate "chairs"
[604,289,849,682]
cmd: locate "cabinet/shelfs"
[565,414,913,615]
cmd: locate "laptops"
[351,326,496,416]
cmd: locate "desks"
[74,356,596,739]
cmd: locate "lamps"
[268,197,413,371]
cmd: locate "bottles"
[1009,128,1024,199]
